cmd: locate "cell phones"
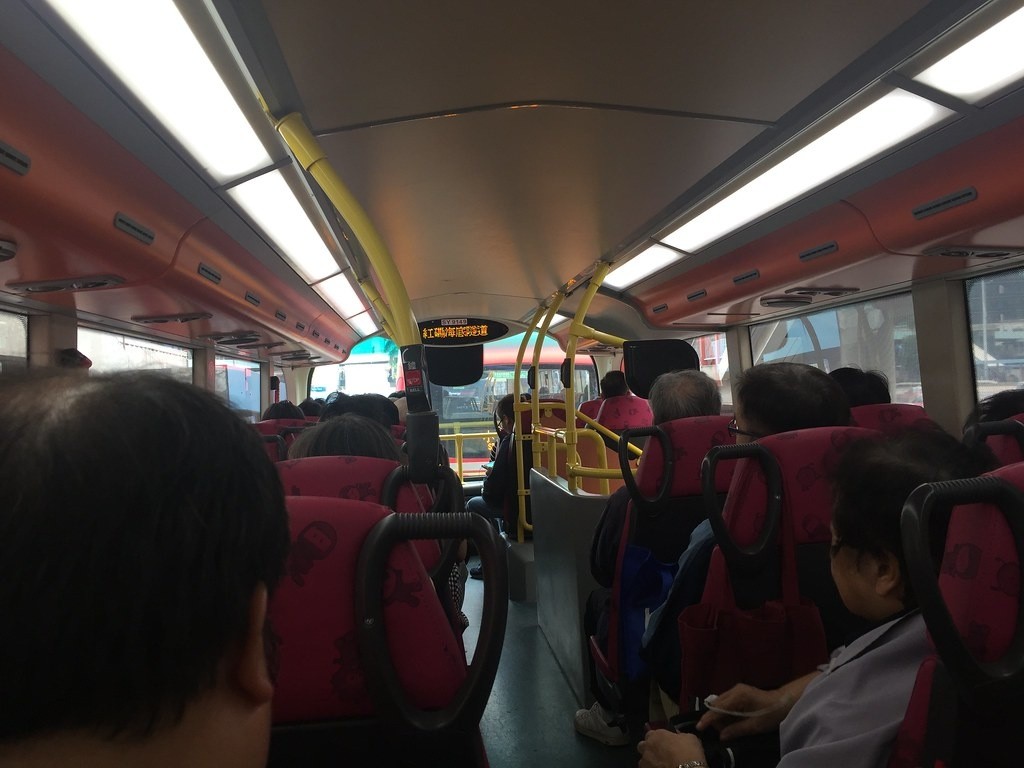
[485,460,495,468]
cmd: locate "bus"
[394,333,724,475]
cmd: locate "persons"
[264,365,1024,768]
[0,370,284,768]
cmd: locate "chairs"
[248,391,1023,768]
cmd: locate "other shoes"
[470,564,483,578]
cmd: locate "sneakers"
[575,700,632,746]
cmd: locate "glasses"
[727,418,762,438]
[497,418,504,429]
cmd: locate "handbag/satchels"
[678,460,829,716]
[701,724,781,768]
